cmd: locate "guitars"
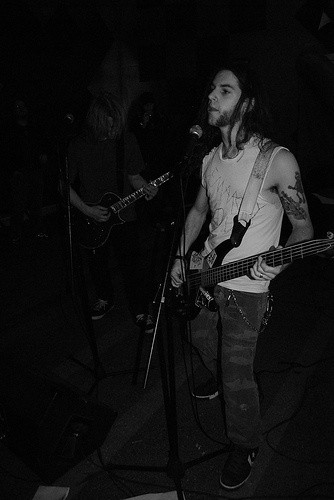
[72,169,173,249]
[161,231,334,321]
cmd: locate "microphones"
[179,124,204,168]
[64,114,75,124]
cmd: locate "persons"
[170,63,314,489]
[56,92,159,333]
[6,97,48,245]
[131,91,159,149]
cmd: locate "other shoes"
[7,232,20,246]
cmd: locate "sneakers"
[136,312,155,333]
[220,446,261,490]
[191,375,224,400]
[90,297,114,320]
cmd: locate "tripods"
[108,238,234,500]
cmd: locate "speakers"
[0,362,118,487]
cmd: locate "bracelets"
[172,256,185,264]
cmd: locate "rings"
[260,273,263,278]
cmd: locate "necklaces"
[225,144,245,159]
